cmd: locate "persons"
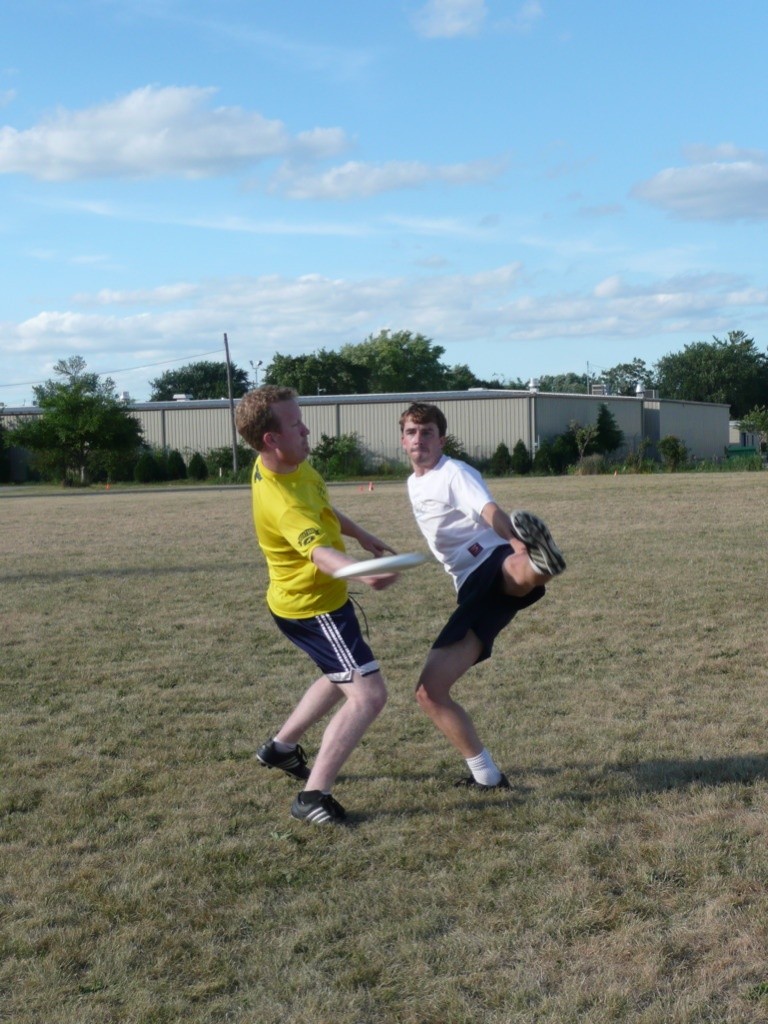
[398,403,566,792]
[233,384,399,827]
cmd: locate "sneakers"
[290,793,346,824]
[255,739,312,780]
[509,510,567,576]
[454,774,510,792]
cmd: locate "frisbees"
[332,552,426,580]
